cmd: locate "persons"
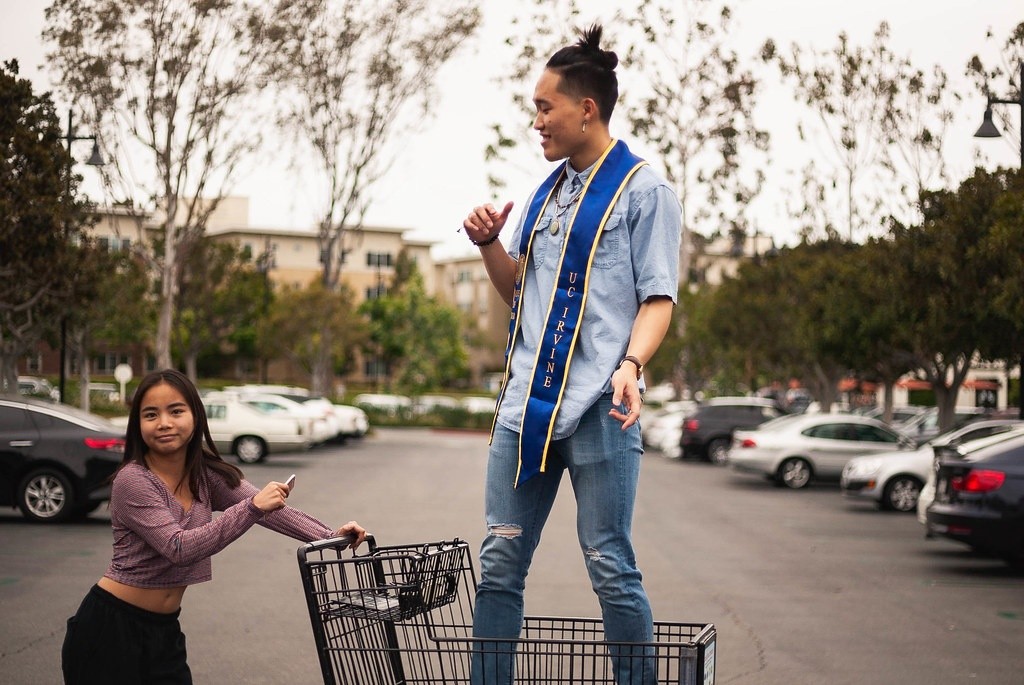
[463,24,681,685]
[61,370,366,685]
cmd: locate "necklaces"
[550,180,582,234]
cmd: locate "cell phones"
[281,475,296,494]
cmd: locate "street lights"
[55,109,106,403]
[969,61,1024,422]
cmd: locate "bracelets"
[472,234,499,246]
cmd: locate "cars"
[727,411,916,492]
[842,417,1024,525]
[634,396,1000,467]
[0,394,131,527]
[5,376,57,400]
[926,434,1024,577]
[112,384,496,464]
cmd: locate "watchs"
[618,357,644,380]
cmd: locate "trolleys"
[295,531,718,684]
